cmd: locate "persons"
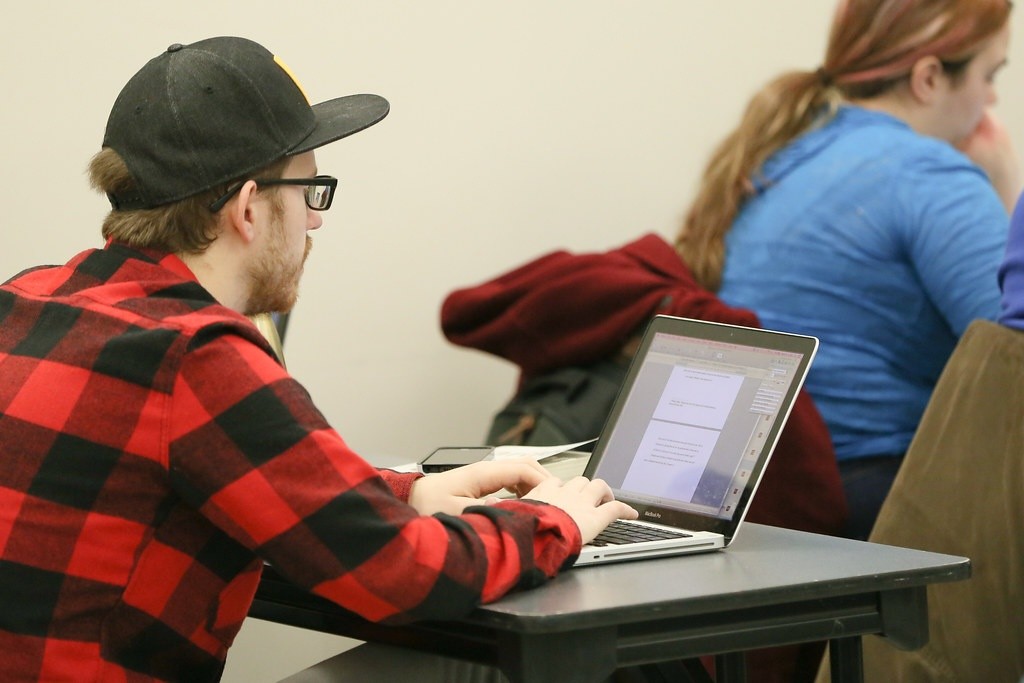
[0,36,640,683]
[670,0,1024,545]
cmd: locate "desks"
[248,523,972,683]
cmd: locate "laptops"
[570,314,820,566]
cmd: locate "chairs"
[815,318,1024,683]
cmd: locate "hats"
[104,36,390,211]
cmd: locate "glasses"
[211,175,338,213]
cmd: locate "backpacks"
[485,358,629,452]
